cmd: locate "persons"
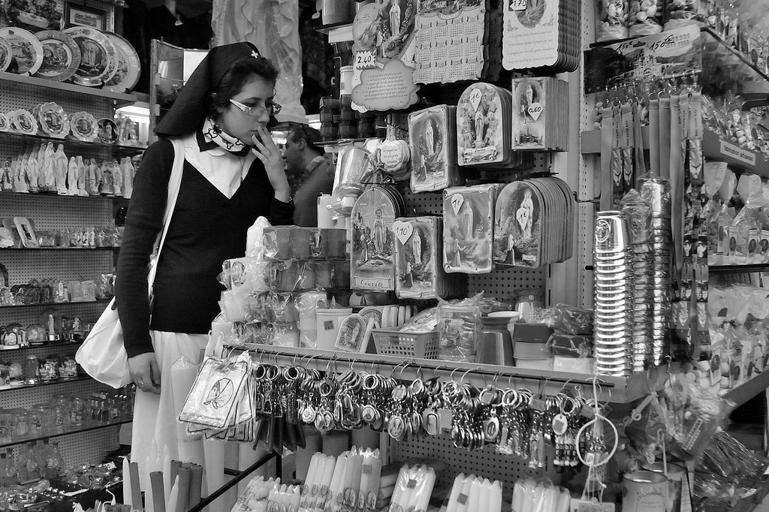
[114,41,295,512]
[282,123,338,227]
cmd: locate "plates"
[33,30,81,83]
[1,26,43,75]
[101,32,142,94]
[0,102,120,144]
[1,37,13,71]
[63,25,117,88]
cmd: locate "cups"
[338,146,370,199]
[641,461,686,511]
[0,279,99,308]
[622,470,670,512]
[68,226,104,247]
[211,226,354,347]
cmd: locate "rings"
[137,382,143,389]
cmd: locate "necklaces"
[301,154,324,177]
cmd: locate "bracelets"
[276,196,293,203]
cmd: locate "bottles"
[24,355,40,385]
[0,385,133,445]
[476,316,516,367]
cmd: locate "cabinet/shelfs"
[582,2,768,415]
[1,68,158,511]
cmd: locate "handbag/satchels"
[74,294,152,389]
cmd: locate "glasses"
[227,97,283,118]
